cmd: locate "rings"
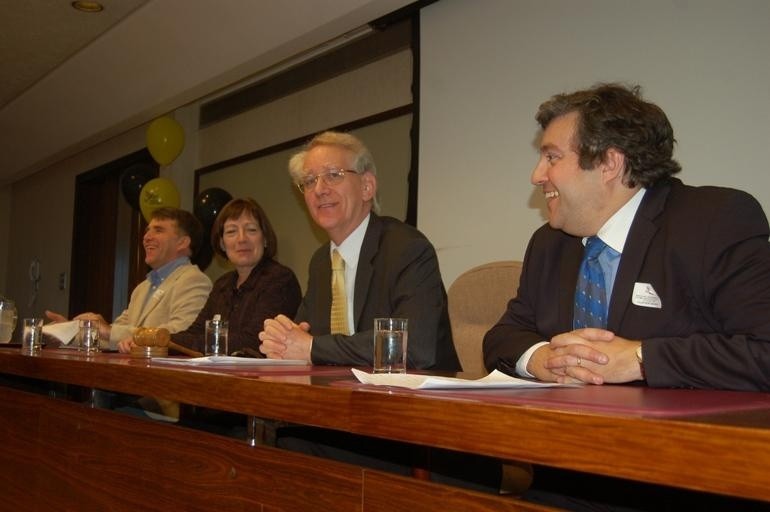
[577,356,582,367]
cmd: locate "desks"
[0,346,770,512]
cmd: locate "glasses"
[297,168,359,194]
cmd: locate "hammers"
[133,327,204,358]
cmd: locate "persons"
[45,207,212,352]
[483,82,769,510]
[115,197,302,435]
[275,131,504,491]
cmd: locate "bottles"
[0,298,19,344]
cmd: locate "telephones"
[28,258,40,281]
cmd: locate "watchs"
[636,344,646,380]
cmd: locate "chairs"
[443,262,535,380]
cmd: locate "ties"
[574,235,608,332]
[328,248,349,337]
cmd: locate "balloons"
[122,165,159,212]
[195,188,234,227]
[140,179,181,225]
[145,116,184,167]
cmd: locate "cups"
[75,320,104,354]
[372,318,411,376]
[22,316,44,349]
[204,320,228,356]
